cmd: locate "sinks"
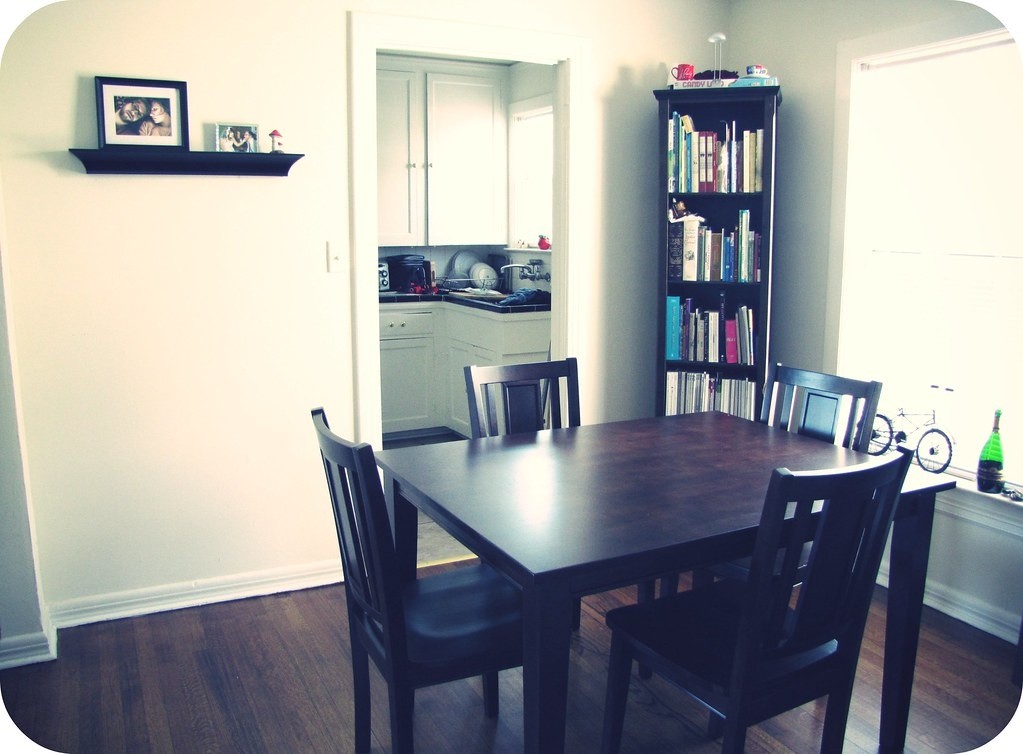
[464,298,551,313]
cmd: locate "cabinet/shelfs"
[377,49,508,247]
[653,86,781,422]
[379,301,551,439]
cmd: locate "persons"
[114,96,150,135]
[219,127,256,152]
[139,98,172,137]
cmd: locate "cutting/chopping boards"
[449,292,508,298]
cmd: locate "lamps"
[708,32,727,79]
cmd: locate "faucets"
[500,258,551,282]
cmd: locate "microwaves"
[378,262,389,291]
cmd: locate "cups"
[746,65,767,74]
[671,64,695,80]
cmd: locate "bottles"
[976,409,1005,493]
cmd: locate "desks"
[373,410,956,754]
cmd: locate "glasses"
[132,102,146,121]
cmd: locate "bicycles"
[857,386,954,473]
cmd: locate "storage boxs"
[673,79,736,90]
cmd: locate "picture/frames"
[216,122,260,152]
[95,76,189,151]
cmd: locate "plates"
[451,250,500,289]
[738,74,770,78]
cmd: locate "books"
[667,111,763,192]
[667,210,761,283]
[665,372,755,422]
[665,293,754,366]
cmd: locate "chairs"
[311,357,916,754]
